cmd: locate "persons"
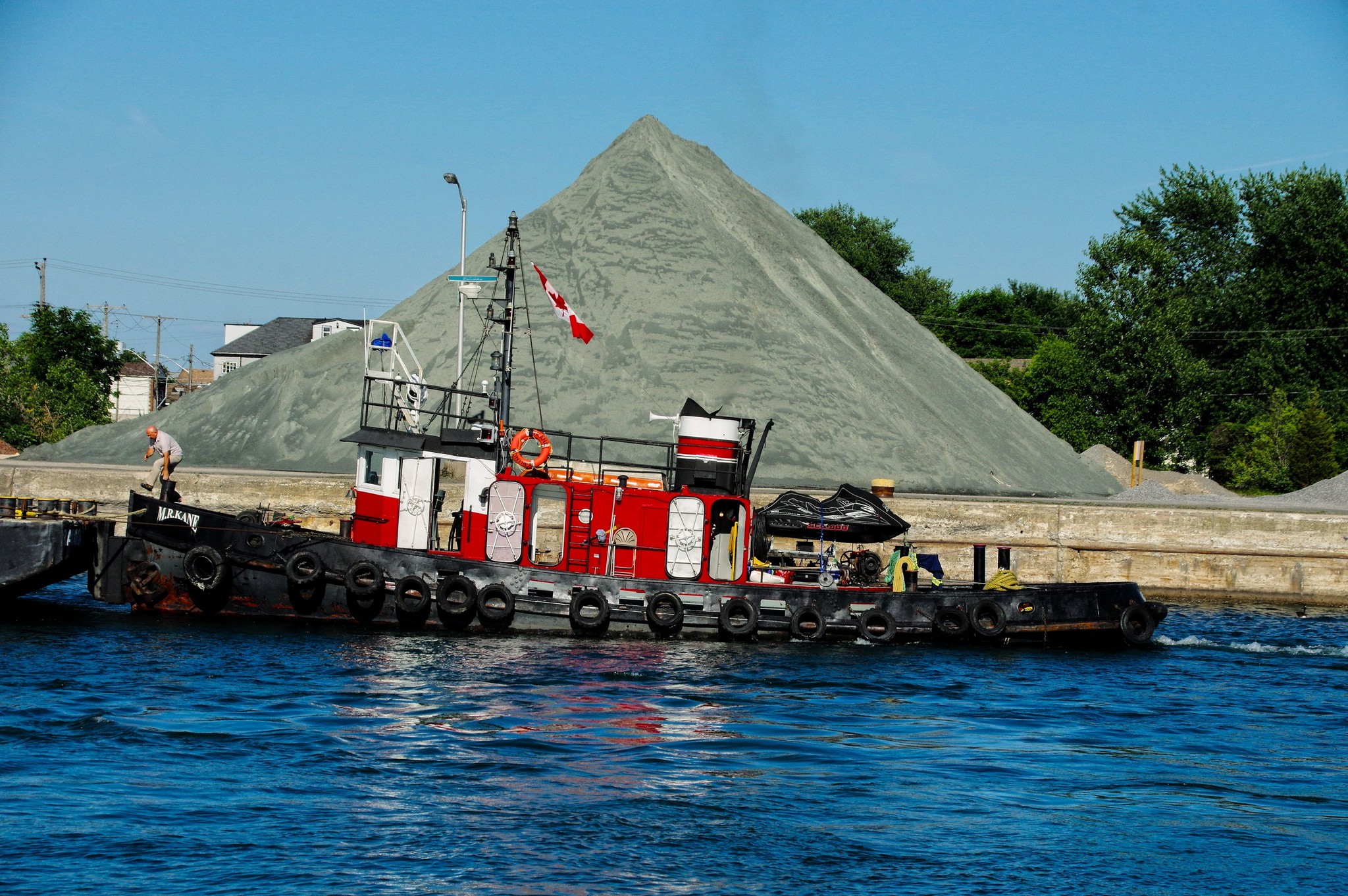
[140,426,183,502]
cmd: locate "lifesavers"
[286,550,324,588]
[436,575,477,615]
[394,576,429,613]
[790,605,827,640]
[510,428,551,469]
[856,607,897,642]
[518,437,538,452]
[345,560,385,597]
[477,583,515,620]
[719,597,759,635]
[569,588,609,628]
[1120,604,1156,644]
[934,606,969,636]
[647,591,684,626]
[184,545,226,591]
[970,599,1007,636]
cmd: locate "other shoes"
[141,483,153,491]
[173,496,181,502]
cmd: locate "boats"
[0,493,116,600]
[87,209,1172,649]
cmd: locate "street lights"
[443,172,468,428]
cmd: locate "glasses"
[147,431,155,436]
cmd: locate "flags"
[531,261,594,344]
